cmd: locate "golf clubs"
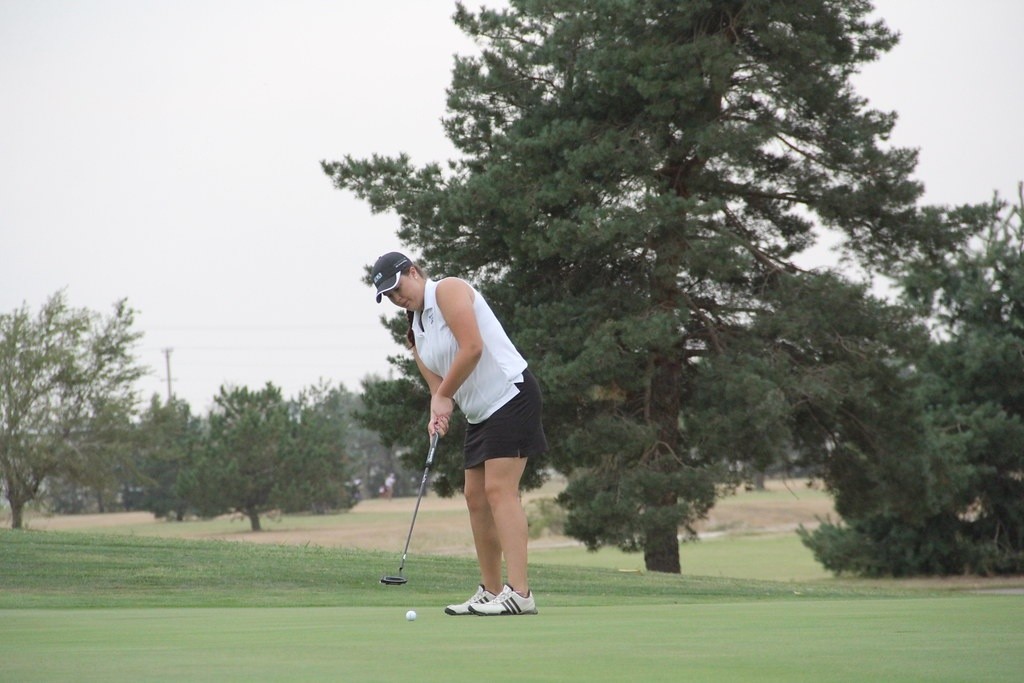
[380,431,438,585]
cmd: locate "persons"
[371,252,549,616]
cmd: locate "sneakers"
[468,585,538,616]
[445,583,496,615]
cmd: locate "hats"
[372,252,413,304]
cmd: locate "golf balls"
[406,611,416,621]
